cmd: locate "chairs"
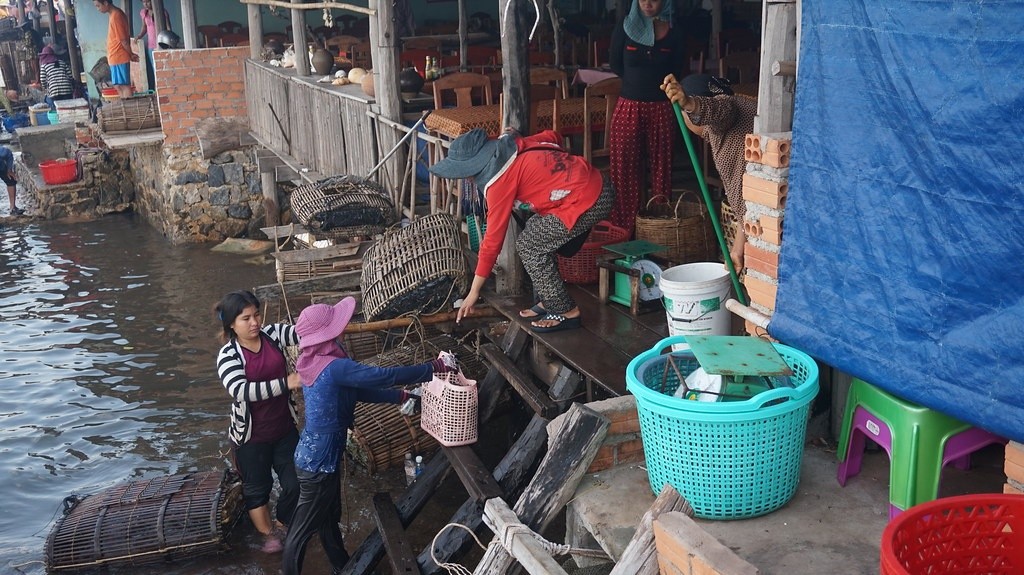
[198,12,758,165]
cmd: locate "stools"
[836,373,1010,526]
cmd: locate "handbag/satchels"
[556,227,592,258]
[420,350,478,447]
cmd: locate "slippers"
[528,310,582,333]
[521,305,548,321]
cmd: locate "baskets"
[626,335,821,520]
[880,493,1024,575]
[38,159,77,184]
[557,220,631,285]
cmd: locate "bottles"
[425,56,440,80]
[362,69,375,96]
[404,453,425,487]
[399,67,424,93]
[264,39,285,55]
[311,48,333,74]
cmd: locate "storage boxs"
[54,100,90,124]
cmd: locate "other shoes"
[262,535,282,554]
[274,525,289,538]
[8,205,25,215]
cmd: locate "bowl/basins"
[0,113,30,133]
[102,85,155,102]
[38,158,77,185]
[30,111,60,125]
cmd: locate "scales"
[679,330,797,406]
[601,240,664,307]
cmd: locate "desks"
[286,32,757,190]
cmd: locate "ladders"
[336,320,581,575]
[470,401,696,575]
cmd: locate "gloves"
[430,354,458,375]
[400,390,421,413]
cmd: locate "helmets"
[157,30,181,49]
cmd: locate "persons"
[0,147,24,215]
[133,0,172,73]
[456,127,615,332]
[660,74,758,277]
[217,290,302,536]
[609,0,689,234]
[0,0,77,107]
[92,0,140,98]
[282,296,459,575]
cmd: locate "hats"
[37,46,58,56]
[0,0,13,8]
[428,126,496,179]
[679,73,735,97]
[295,295,356,349]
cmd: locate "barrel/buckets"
[659,262,731,352]
